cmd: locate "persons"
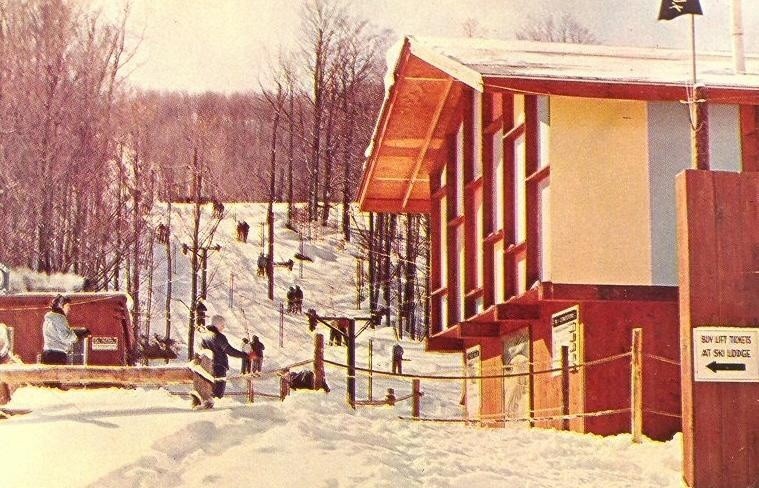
[391,341,404,373]
[212,199,219,218]
[339,314,349,346]
[157,222,166,244]
[200,315,248,399]
[293,285,303,315]
[328,319,341,347]
[237,220,243,241]
[39,293,91,391]
[240,331,251,375]
[242,219,250,242]
[249,335,265,378]
[217,201,224,218]
[256,252,266,276]
[287,286,296,314]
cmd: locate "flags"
[657,0,704,21]
